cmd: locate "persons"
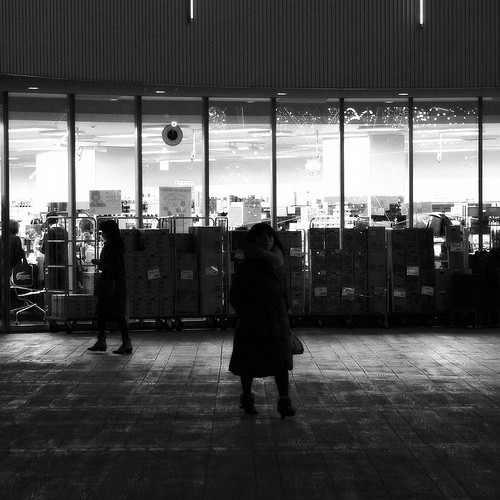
[41,211,68,291]
[79,218,93,235]
[229,224,296,420]
[87,219,133,354]
[7,222,26,268]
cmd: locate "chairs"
[10,264,48,324]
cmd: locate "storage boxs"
[50,225,437,319]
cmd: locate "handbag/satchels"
[291,331,303,354]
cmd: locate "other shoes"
[112,345,132,353]
[89,343,108,351]
[241,395,255,411]
[276,399,295,418]
